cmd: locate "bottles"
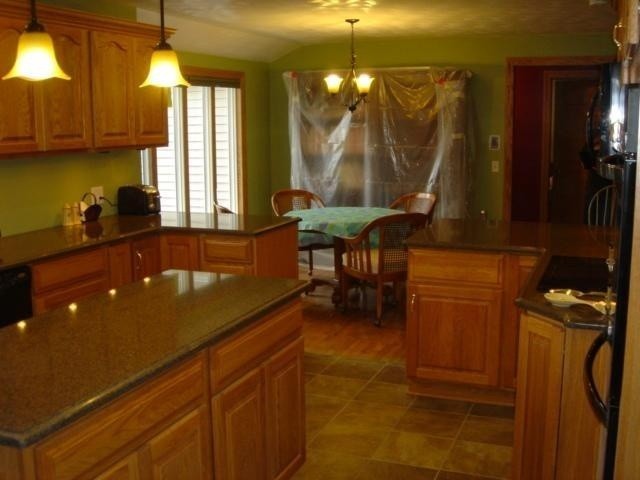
[64,202,70,227]
[72,201,80,225]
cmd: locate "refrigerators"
[580,83,639,480]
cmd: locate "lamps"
[133,0,195,93]
[323,17,376,113]
[1,0,78,84]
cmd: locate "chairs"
[211,198,241,216]
[382,191,443,225]
[329,208,428,328]
[269,185,333,277]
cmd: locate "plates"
[549,288,584,298]
[544,292,576,308]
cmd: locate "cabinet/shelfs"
[89,11,178,156]
[404,243,606,480]
[29,232,257,311]
[1,0,96,158]
[1,264,313,480]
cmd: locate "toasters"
[118,183,161,217]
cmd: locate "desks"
[275,205,411,307]
